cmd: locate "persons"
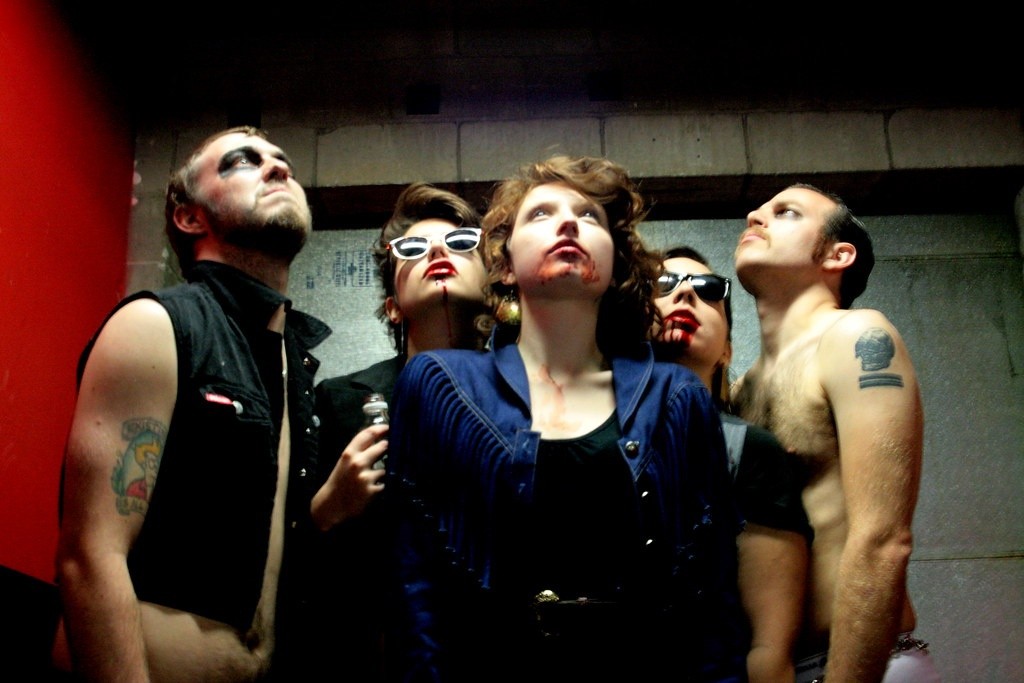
[55,122,936,683]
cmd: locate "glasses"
[647,270,731,303]
[384,227,483,260]
